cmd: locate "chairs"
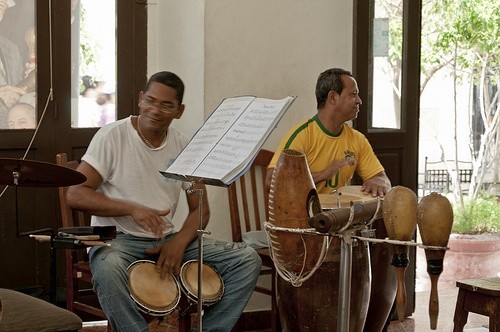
[55,153,204,332]
[227,148,282,332]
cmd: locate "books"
[164,95,294,185]
[317,185,380,210]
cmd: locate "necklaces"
[138,115,169,149]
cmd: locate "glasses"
[137,96,179,112]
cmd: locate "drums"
[178,260,224,315]
[336,185,399,332]
[276,194,372,332]
[127,260,180,323]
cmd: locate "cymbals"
[0,158,87,187]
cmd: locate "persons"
[66,71,262,332]
[266,68,390,197]
[0,0,119,129]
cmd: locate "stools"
[452,277,500,332]
